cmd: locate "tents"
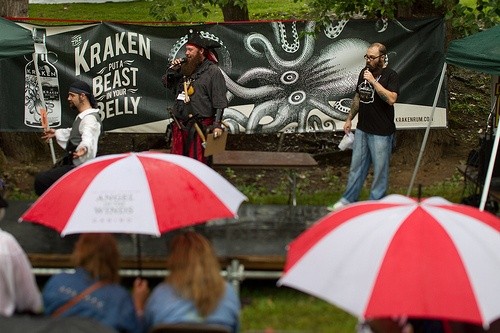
[406,23,500,212]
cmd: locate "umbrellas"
[278,194,500,333]
[18,151,250,238]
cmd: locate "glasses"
[364,54,386,61]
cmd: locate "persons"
[41,233,150,333]
[356,315,500,333]
[162,35,226,164]
[34,80,105,194]
[145,231,242,333]
[325,42,400,214]
[0,198,43,317]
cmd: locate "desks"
[212,152,317,207]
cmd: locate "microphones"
[168,56,188,69]
[364,66,369,87]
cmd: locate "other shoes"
[326,201,344,210]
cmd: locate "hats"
[188,33,209,50]
[69,80,91,97]
